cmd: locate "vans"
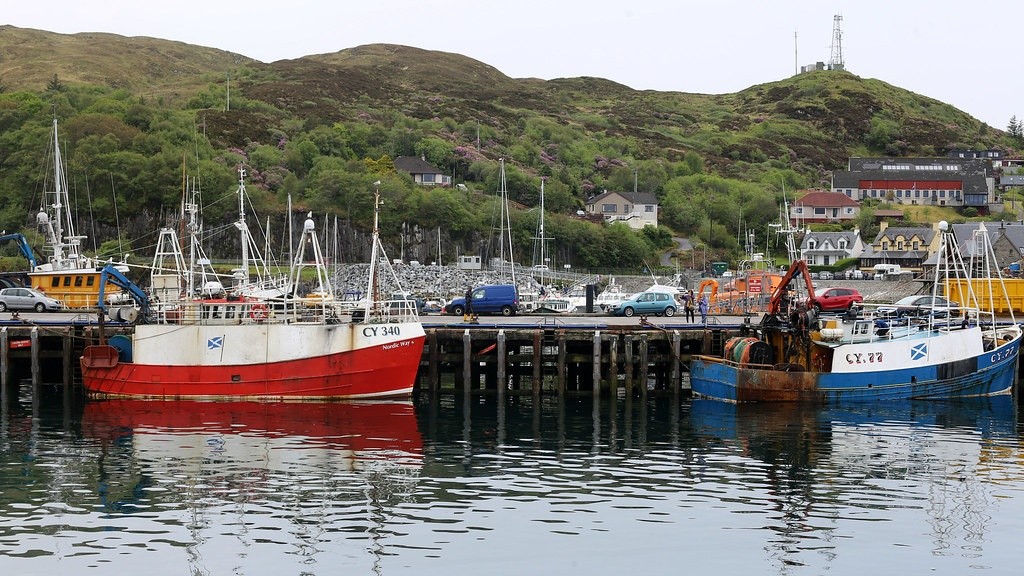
[445,284,521,317]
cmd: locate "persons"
[698,293,708,323]
[680,290,695,323]
[539,287,545,295]
[465,286,473,314]
[848,271,869,280]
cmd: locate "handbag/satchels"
[689,305,695,311]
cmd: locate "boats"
[687,177,1024,407]
[23,106,428,401]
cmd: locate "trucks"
[874,263,900,280]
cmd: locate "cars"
[875,294,960,321]
[609,291,678,319]
[0,287,63,313]
[404,294,447,313]
[533,264,549,273]
[806,269,875,281]
[793,287,865,317]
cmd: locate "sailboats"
[474,155,712,316]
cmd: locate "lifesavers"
[34,285,45,294]
[250,305,267,322]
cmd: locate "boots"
[463,313,469,322]
[470,314,473,320]
